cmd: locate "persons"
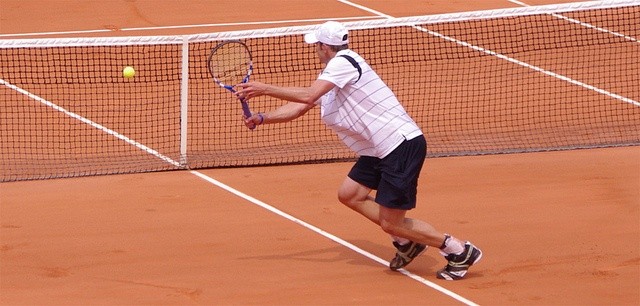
[233,21,483,281]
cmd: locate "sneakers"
[389,242,427,272]
[437,242,482,280]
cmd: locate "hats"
[306,21,349,45]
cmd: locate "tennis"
[123,66,135,79]
